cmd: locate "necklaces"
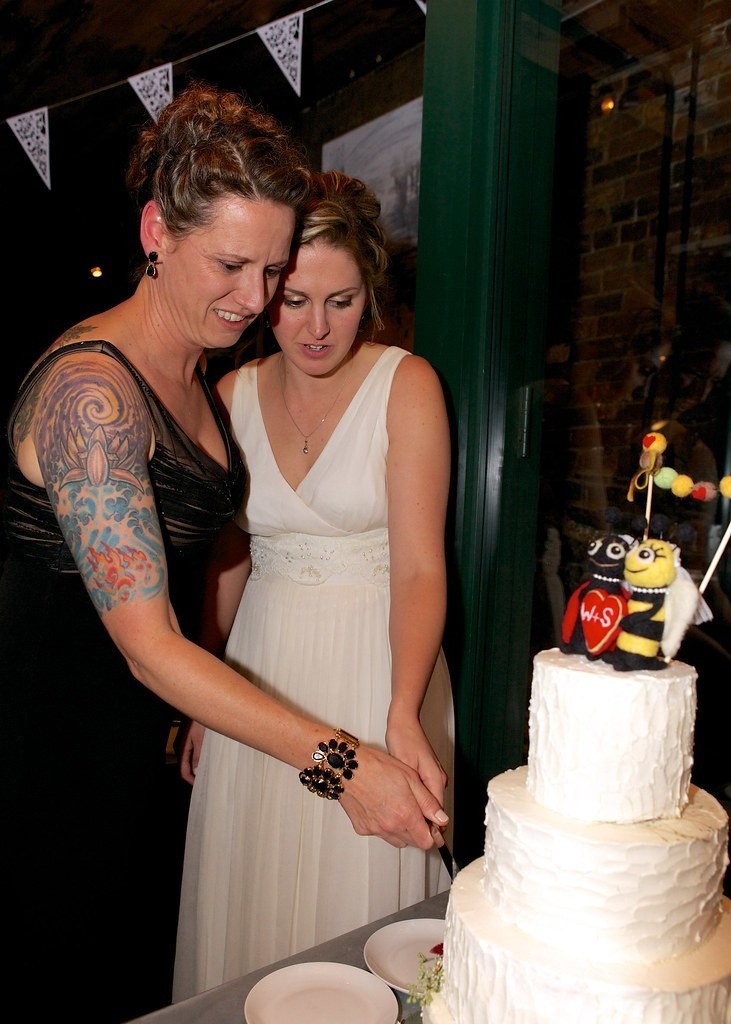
[279,344,355,456]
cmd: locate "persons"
[542,277,731,629]
[0,87,450,1024]
[172,172,454,1006]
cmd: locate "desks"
[132,890,450,1024]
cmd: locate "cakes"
[422,530,731,1024]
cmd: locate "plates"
[244,962,398,1024]
[364,918,445,996]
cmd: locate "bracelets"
[299,729,359,801]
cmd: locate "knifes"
[428,823,460,882]
